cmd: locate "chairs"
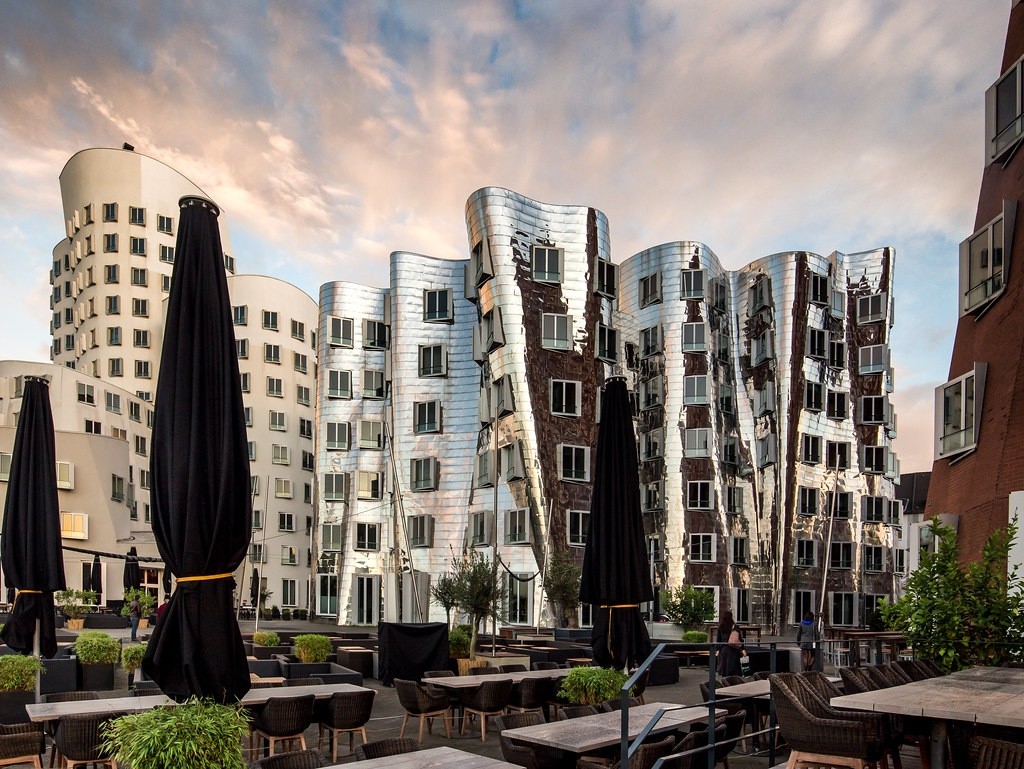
[0,631,1024,769]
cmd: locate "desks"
[714,677,846,755]
[829,665,1024,769]
[501,701,728,769]
[315,745,526,769]
[25,683,378,769]
[500,627,556,640]
[419,667,652,736]
[823,627,870,659]
[844,631,909,668]
[873,635,909,660]
[710,626,762,647]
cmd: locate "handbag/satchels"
[813,643,816,648]
[728,625,742,649]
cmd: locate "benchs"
[55,608,158,629]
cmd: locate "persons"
[129,595,142,641]
[797,610,817,670]
[715,611,748,679]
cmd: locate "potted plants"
[120,585,158,630]
[55,587,98,631]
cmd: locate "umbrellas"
[140,195,253,702]
[251,567,259,607]
[0,375,69,703]
[6,546,173,606]
[578,375,658,673]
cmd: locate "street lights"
[648,534,660,623]
